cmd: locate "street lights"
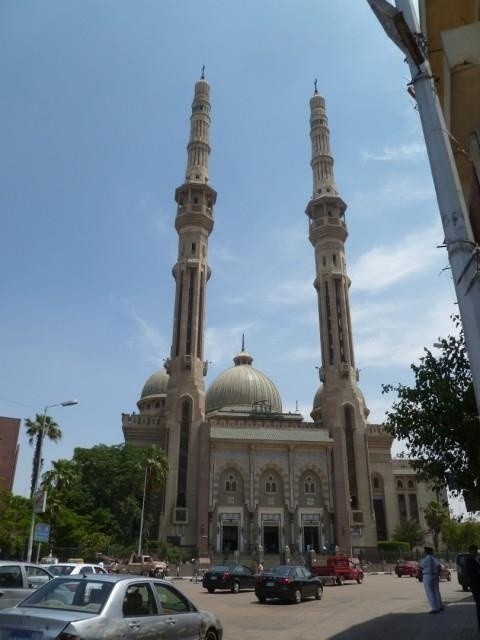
[138,456,159,556]
[24,400,78,561]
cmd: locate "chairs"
[86,589,108,606]
[124,593,151,615]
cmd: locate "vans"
[457,551,480,591]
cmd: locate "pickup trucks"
[311,556,366,586]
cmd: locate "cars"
[202,563,258,593]
[416,562,451,582]
[0,553,167,612]
[254,565,323,604]
[394,560,419,576]
[0,571,223,639]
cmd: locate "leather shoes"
[428,606,445,614]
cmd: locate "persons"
[420,546,444,614]
[257,560,264,574]
[466,544,480,626]
[99,559,121,574]
